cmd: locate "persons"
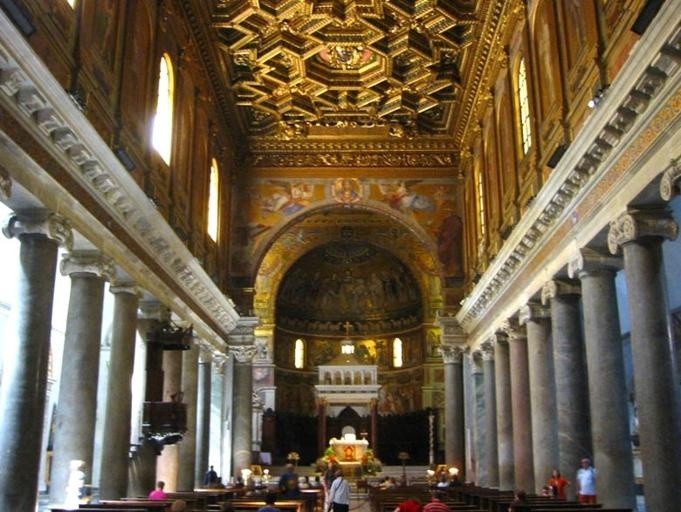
[540,468,572,499]
[377,475,397,489]
[149,480,168,500]
[437,474,450,486]
[421,492,451,512]
[508,488,531,512]
[204,461,350,512]
[395,491,422,512]
[575,458,597,505]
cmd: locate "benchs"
[367,480,605,511]
[48,478,327,510]
